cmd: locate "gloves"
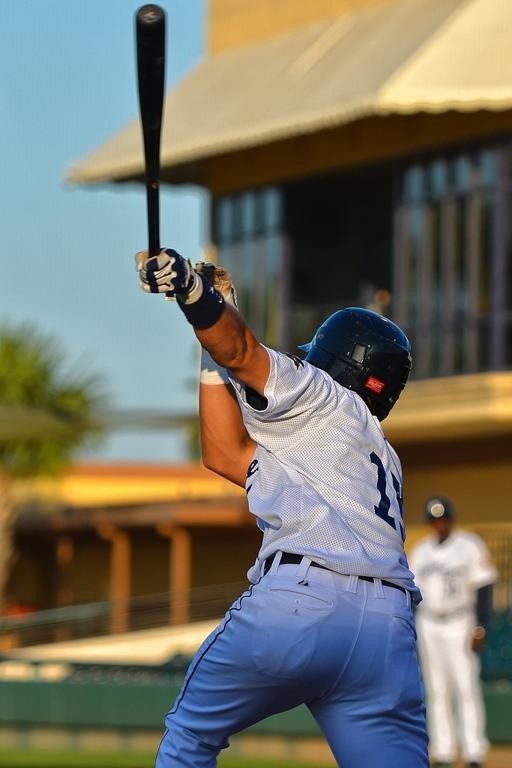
[134,248,239,386]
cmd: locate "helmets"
[297,307,413,422]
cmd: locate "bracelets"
[198,344,235,387]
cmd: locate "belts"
[263,550,406,595]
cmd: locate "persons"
[133,241,432,767]
[408,496,502,768]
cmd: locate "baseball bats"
[135,3,166,255]
